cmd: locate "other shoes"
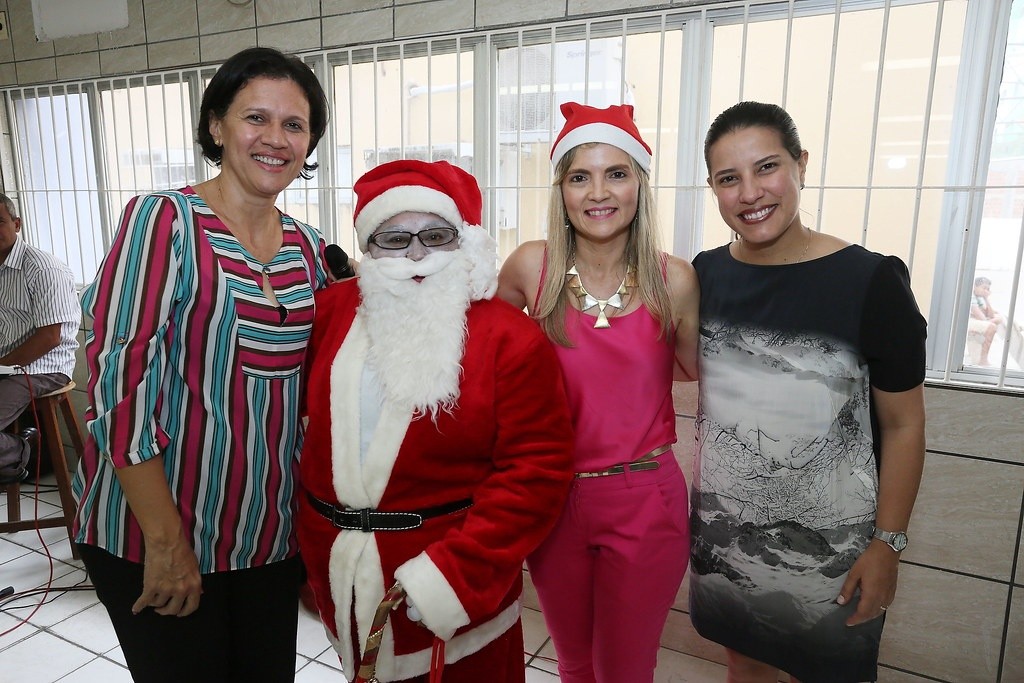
[0,468,29,486]
[20,427,41,449]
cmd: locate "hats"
[550,101,653,180]
[353,160,482,255]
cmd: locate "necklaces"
[565,240,637,331]
[737,227,814,265]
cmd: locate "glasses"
[368,227,458,250]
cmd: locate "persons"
[966,277,1003,367]
[0,191,82,490]
[497,101,704,682]
[293,159,577,683]
[68,45,353,683]
[662,99,929,683]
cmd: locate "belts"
[305,490,472,533]
[575,442,672,479]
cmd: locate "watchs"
[867,527,909,553]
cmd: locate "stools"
[0,380,86,560]
